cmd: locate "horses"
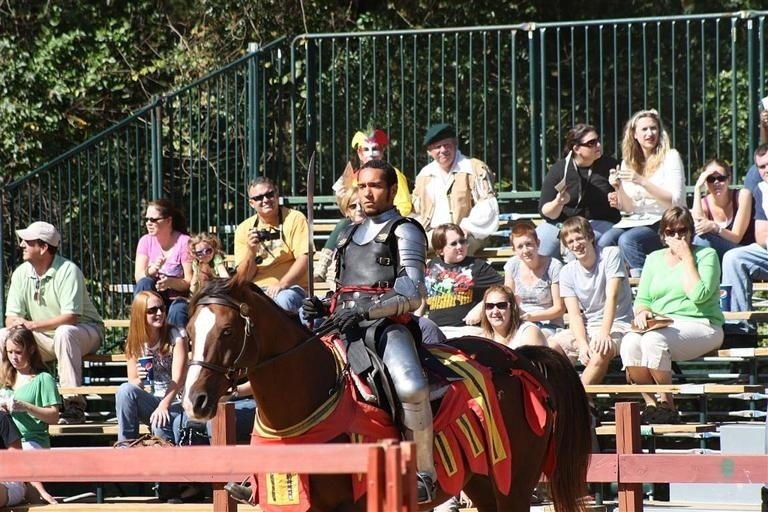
[180,257,595,512]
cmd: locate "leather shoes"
[224,481,252,501]
[415,473,433,500]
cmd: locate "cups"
[720,283,733,313]
[137,358,155,385]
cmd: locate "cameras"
[257,229,270,241]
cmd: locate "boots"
[313,247,334,282]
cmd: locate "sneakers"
[57,406,85,424]
[640,402,678,423]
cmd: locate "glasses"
[34,280,40,301]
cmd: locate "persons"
[225,160,438,503]
[322,123,503,339]
[0,219,105,506]
[481,107,768,426]
[115,177,317,446]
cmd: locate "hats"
[15,220,60,248]
[422,123,456,147]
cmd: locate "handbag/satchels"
[177,427,210,445]
[631,316,675,334]
[114,434,175,448]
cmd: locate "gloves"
[333,306,364,334]
[301,295,330,319]
